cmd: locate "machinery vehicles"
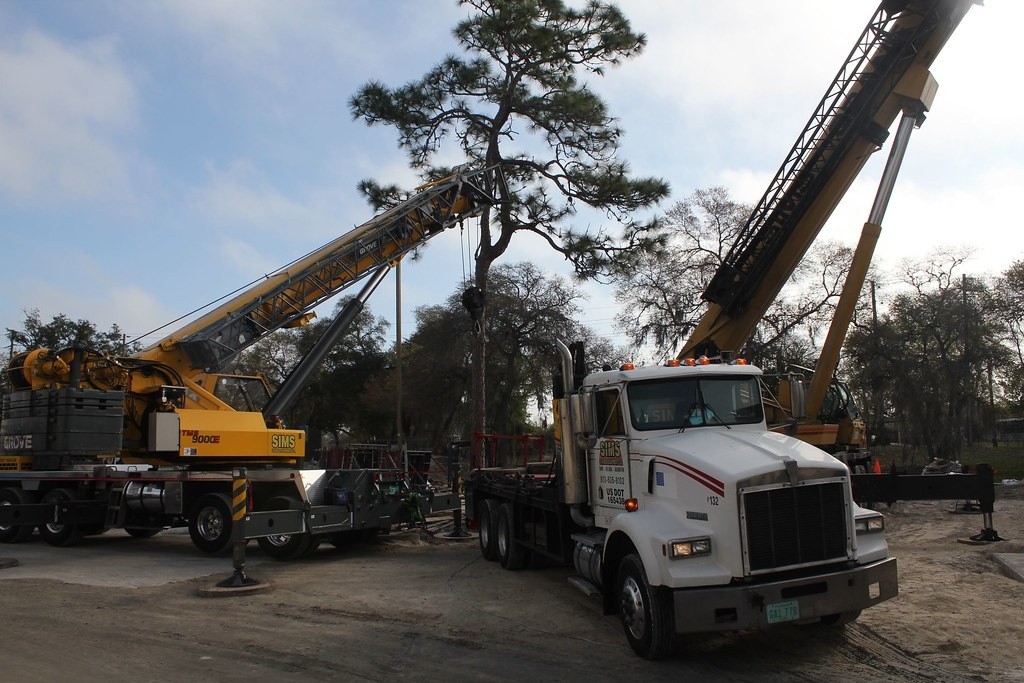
[1,162,517,602]
[468,0,1004,668]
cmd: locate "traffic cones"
[873,457,882,474]
